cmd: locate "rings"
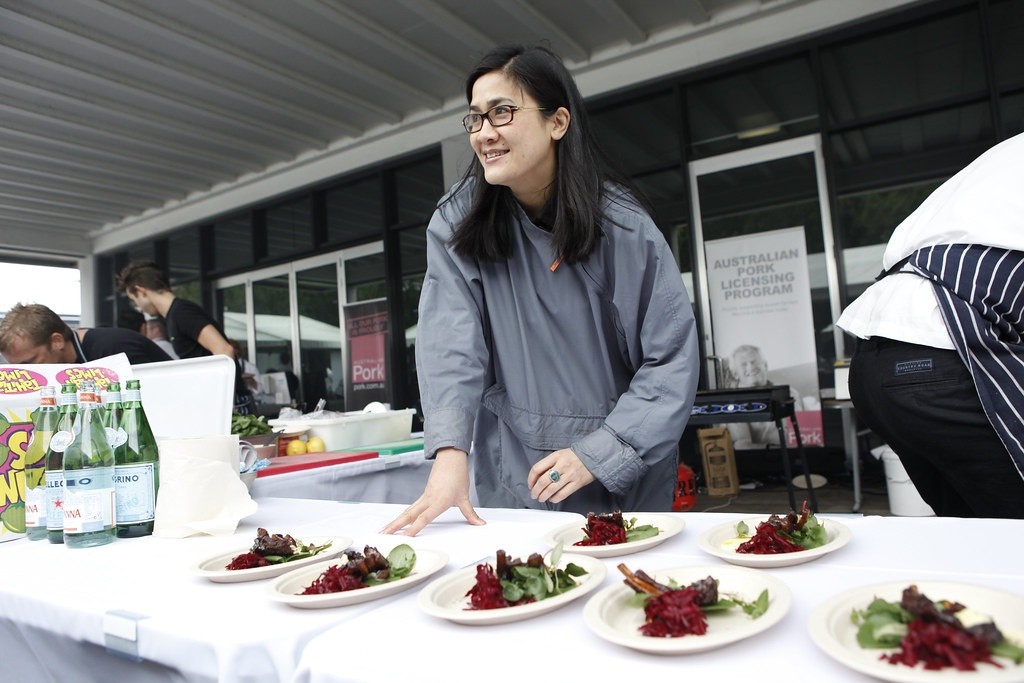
[547,469,560,483]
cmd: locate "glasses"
[462,103,553,134]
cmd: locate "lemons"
[286,437,325,456]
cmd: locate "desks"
[249,427,474,507]
[1,493,1024,683]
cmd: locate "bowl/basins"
[253,443,276,460]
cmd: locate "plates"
[195,536,353,583]
[418,553,606,626]
[267,547,449,609]
[272,426,311,437]
[544,512,684,558]
[698,516,852,568]
[812,580,1024,683]
[582,566,791,654]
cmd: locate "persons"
[381,48,700,536]
[97,260,300,426]
[0,303,174,364]
[848,132,1024,519]
[731,344,805,431]
[269,353,336,408]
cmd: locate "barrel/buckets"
[881,445,935,517]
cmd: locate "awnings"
[222,311,341,348]
[405,324,417,347]
[681,244,891,306]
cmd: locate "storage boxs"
[698,426,740,496]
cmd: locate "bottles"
[25,380,160,548]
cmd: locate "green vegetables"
[262,539,417,588]
[851,594,1023,666]
[583,518,659,543]
[501,541,588,602]
[635,575,770,619]
[735,512,828,551]
[231,410,273,437]
